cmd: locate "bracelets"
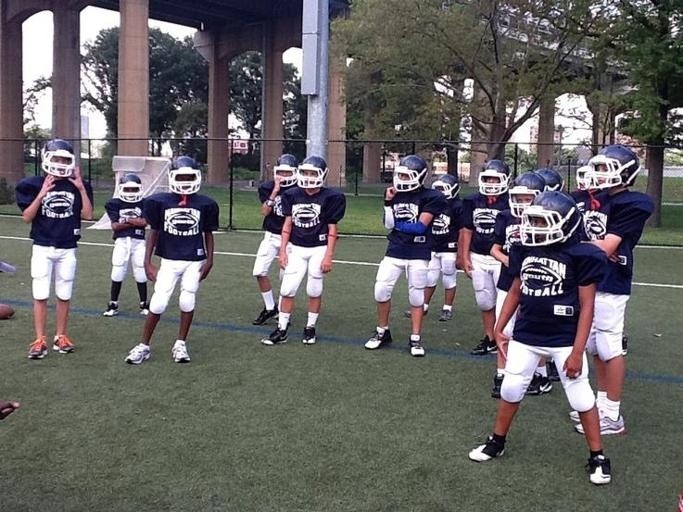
[264,197,276,208]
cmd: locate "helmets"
[478,145,641,246]
[393,155,427,192]
[274,154,329,189]
[118,174,142,204]
[432,174,460,200]
[167,156,201,195]
[41,139,75,177]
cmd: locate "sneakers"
[469,436,504,461]
[253,306,316,345]
[410,334,425,356]
[439,309,453,321]
[140,301,150,315]
[471,335,559,397]
[28,335,74,359]
[172,340,190,362]
[365,327,391,349]
[103,301,118,316]
[569,408,625,435]
[125,343,151,364]
[585,455,611,484]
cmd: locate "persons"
[561,145,656,439]
[124,153,220,364]
[103,173,152,318]
[252,154,348,344]
[490,173,553,401]
[362,142,655,356]
[468,189,611,485]
[14,139,93,362]
[0,303,15,321]
[0,399,20,421]
